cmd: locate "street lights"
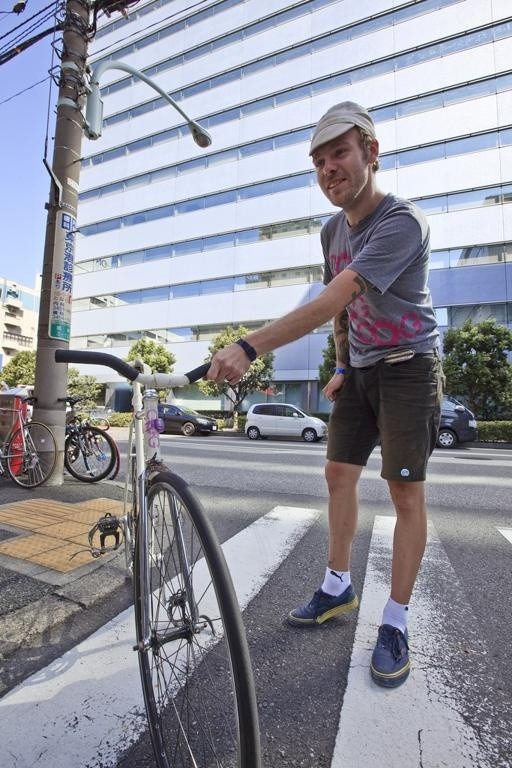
[51,60,213,175]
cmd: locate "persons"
[201,102,445,690]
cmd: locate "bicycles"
[56,394,120,483]
[1,395,57,489]
[55,348,261,767]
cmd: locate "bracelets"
[236,339,257,361]
[335,367,346,374]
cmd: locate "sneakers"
[370,624,410,687]
[288,584,358,627]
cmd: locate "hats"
[308,101,379,167]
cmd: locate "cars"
[244,402,328,443]
[157,403,218,436]
[437,394,477,449]
